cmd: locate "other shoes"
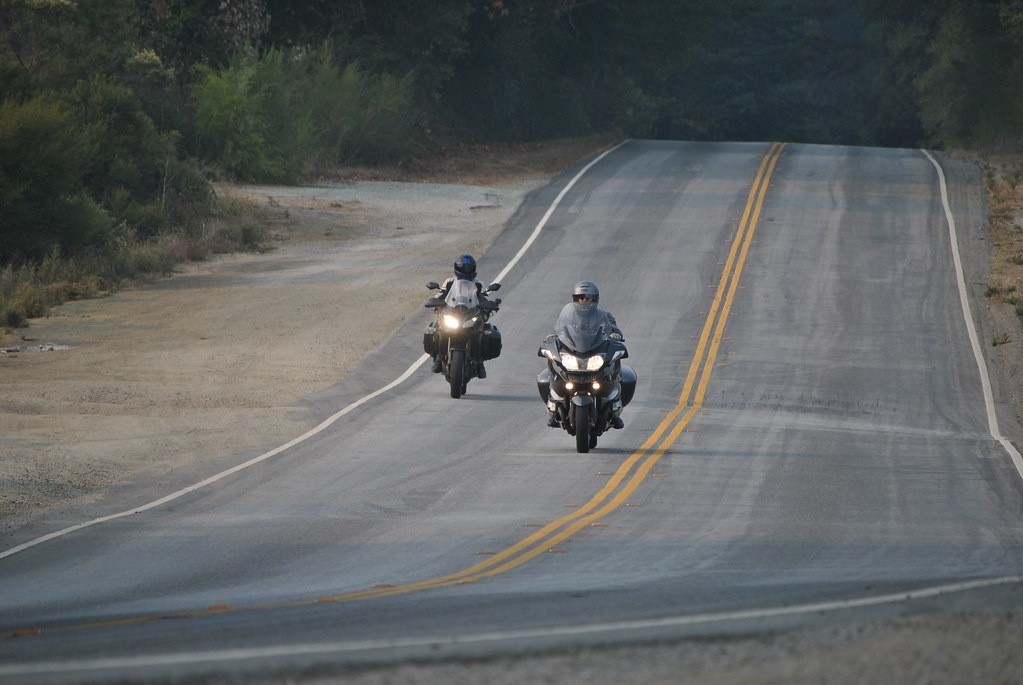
[611,416,624,429]
[546,415,560,427]
[477,361,487,378]
[432,362,442,373]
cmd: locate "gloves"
[610,332,622,340]
[545,331,557,342]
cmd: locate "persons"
[546,280,625,429]
[427,254,498,379]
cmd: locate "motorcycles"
[536,302,638,453]
[423,281,503,398]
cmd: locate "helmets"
[453,253,478,281]
[572,279,600,312]
